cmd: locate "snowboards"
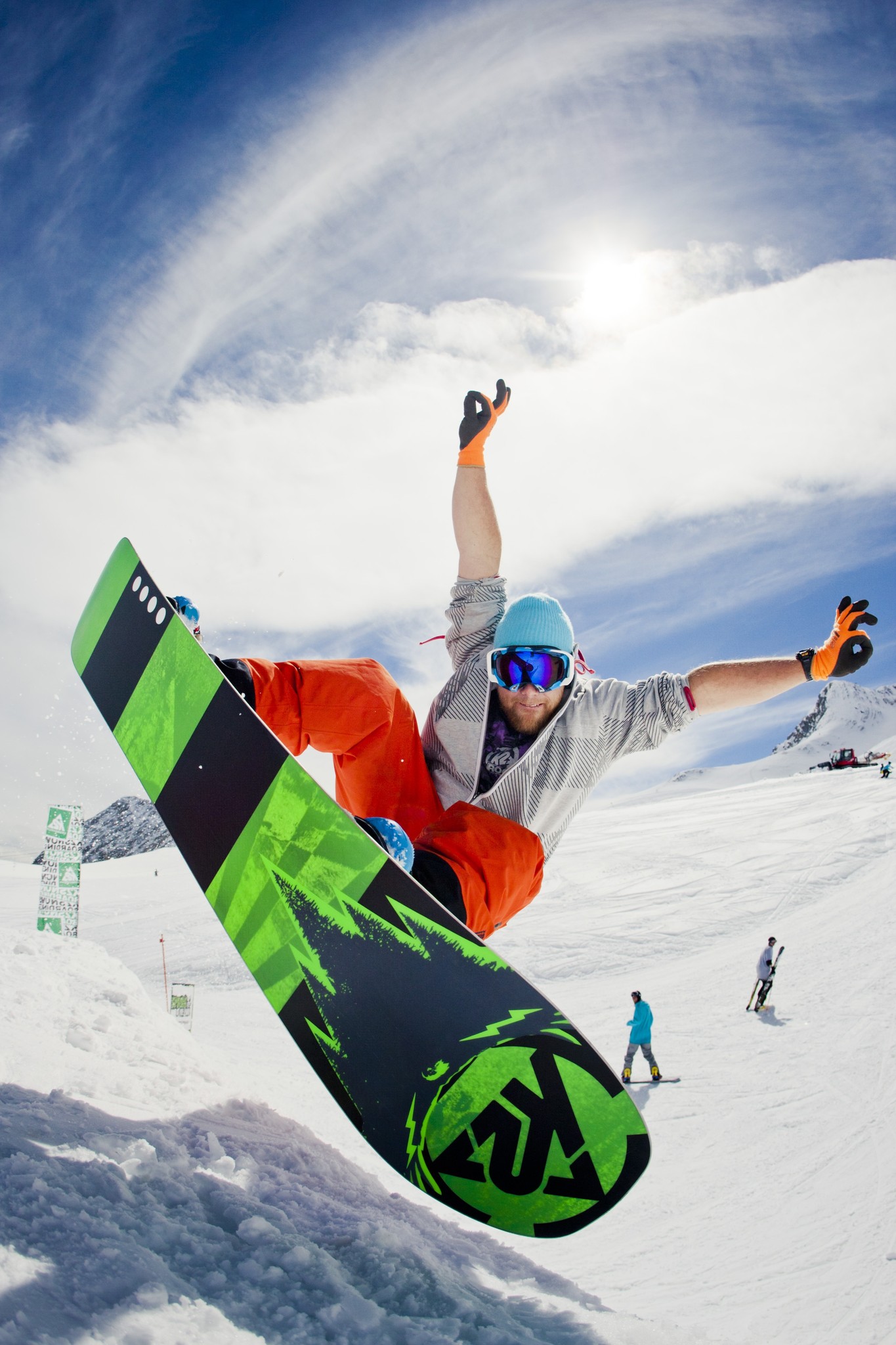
[627,1076,680,1083]
[70,538,651,1239]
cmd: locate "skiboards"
[746,946,784,1012]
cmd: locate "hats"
[768,937,775,942]
[493,595,576,657]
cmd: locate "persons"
[619,990,662,1085]
[161,380,879,943]
[755,936,777,1011]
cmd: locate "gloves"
[811,596,878,681]
[771,967,775,974]
[457,380,512,471]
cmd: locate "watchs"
[794,648,818,682]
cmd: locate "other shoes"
[757,1005,765,1010]
[355,815,417,876]
[166,596,199,643]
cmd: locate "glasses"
[485,647,572,692]
[771,940,776,943]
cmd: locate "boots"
[651,1066,662,1080]
[622,1068,631,1083]
